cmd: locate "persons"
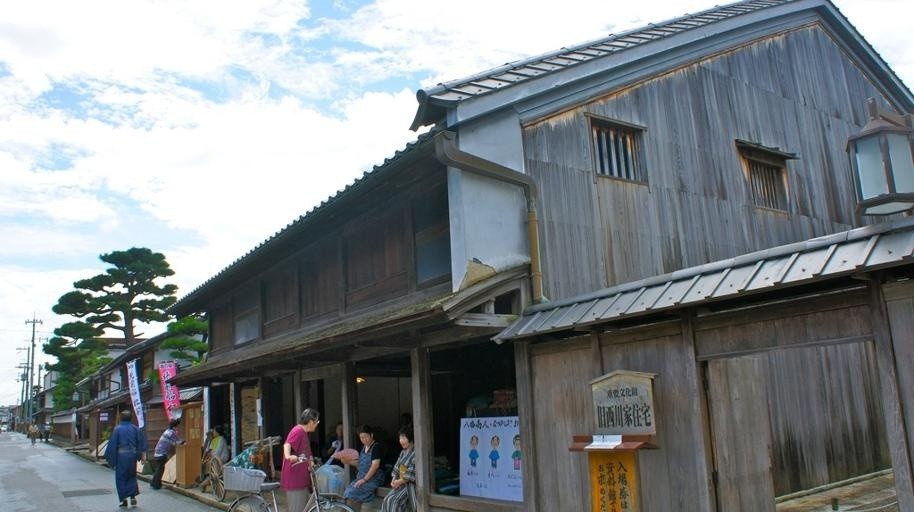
[279,407,320,511]
[208,425,229,463]
[488,435,500,467]
[313,420,344,500]
[149,417,187,490]
[511,434,523,469]
[102,409,148,506]
[379,424,417,511]
[339,423,389,512]
[201,427,213,464]
[27,419,38,443]
[468,435,479,467]
[43,421,51,443]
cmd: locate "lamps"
[72,388,80,401]
[844,94,914,219]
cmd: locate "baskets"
[315,465,346,497]
[222,465,267,494]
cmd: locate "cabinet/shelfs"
[175,439,202,489]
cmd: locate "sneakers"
[131,500,137,506]
[120,500,127,507]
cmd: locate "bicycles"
[224,456,356,512]
